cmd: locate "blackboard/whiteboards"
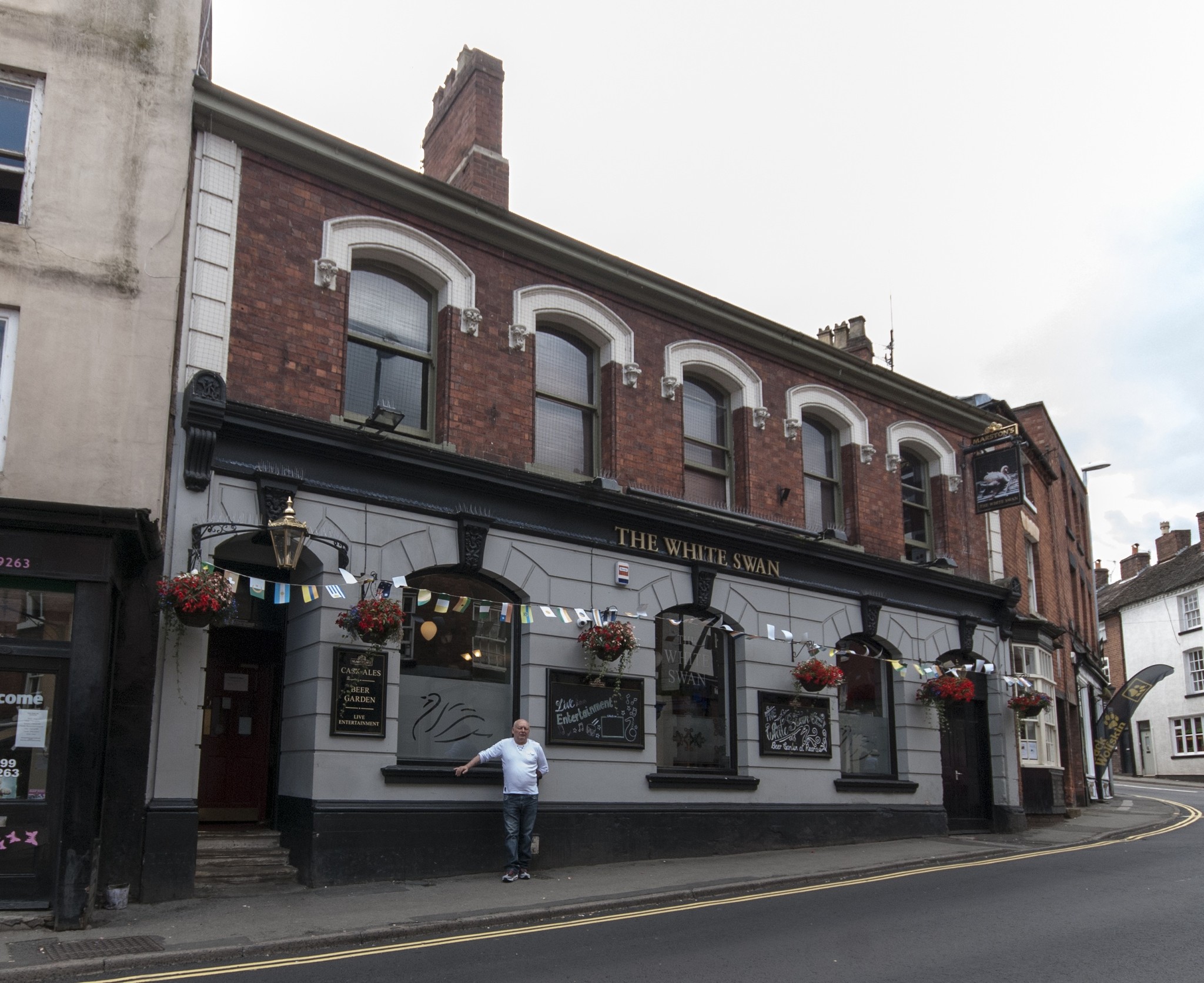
[757,688,832,760]
[546,667,648,750]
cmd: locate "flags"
[197,562,1030,690]
[1093,665,1173,780]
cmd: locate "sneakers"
[518,868,530,879]
[502,869,518,882]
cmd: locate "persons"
[453,719,549,881]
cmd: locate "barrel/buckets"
[103,882,130,910]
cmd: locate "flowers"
[578,621,640,719]
[158,570,237,705]
[335,599,405,718]
[1007,690,1053,747]
[916,677,975,740]
[790,657,844,722]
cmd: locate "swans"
[984,465,1011,485]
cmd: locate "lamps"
[814,528,848,542]
[268,497,309,569]
[579,478,621,492]
[913,557,958,569]
[357,405,405,433]
